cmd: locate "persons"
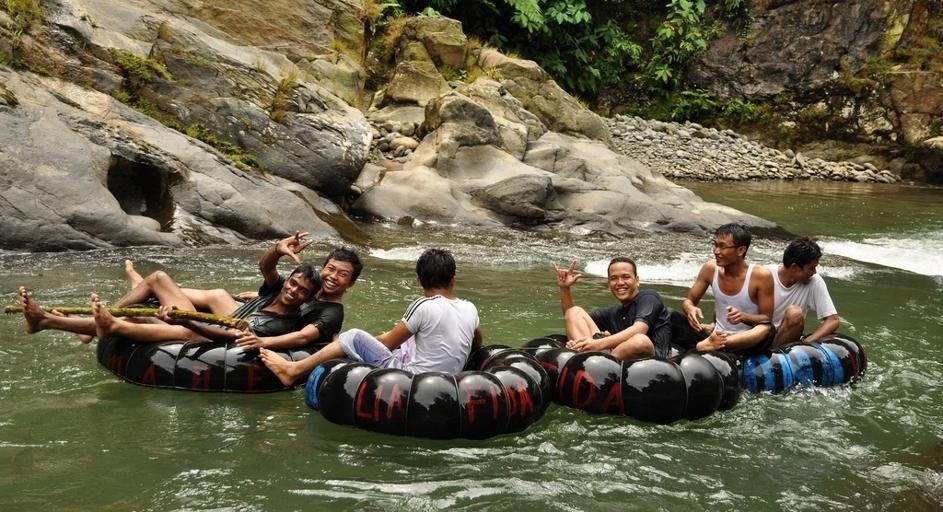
[699,237,839,350]
[259,249,483,386]
[551,257,672,361]
[90,246,362,352]
[20,230,320,343]
[666,223,776,357]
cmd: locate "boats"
[95,328,325,393]
[684,335,869,391]
[307,344,552,437]
[526,334,741,424]
